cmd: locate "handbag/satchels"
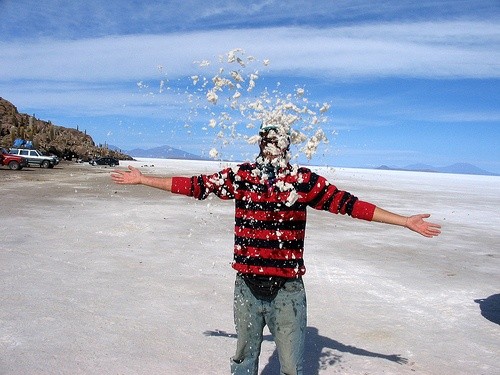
[237,272,287,303]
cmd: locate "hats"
[259,123,291,135]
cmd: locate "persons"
[110,122,442,375]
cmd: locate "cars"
[89,157,120,167]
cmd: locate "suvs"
[0,147,28,171]
[7,148,63,168]
